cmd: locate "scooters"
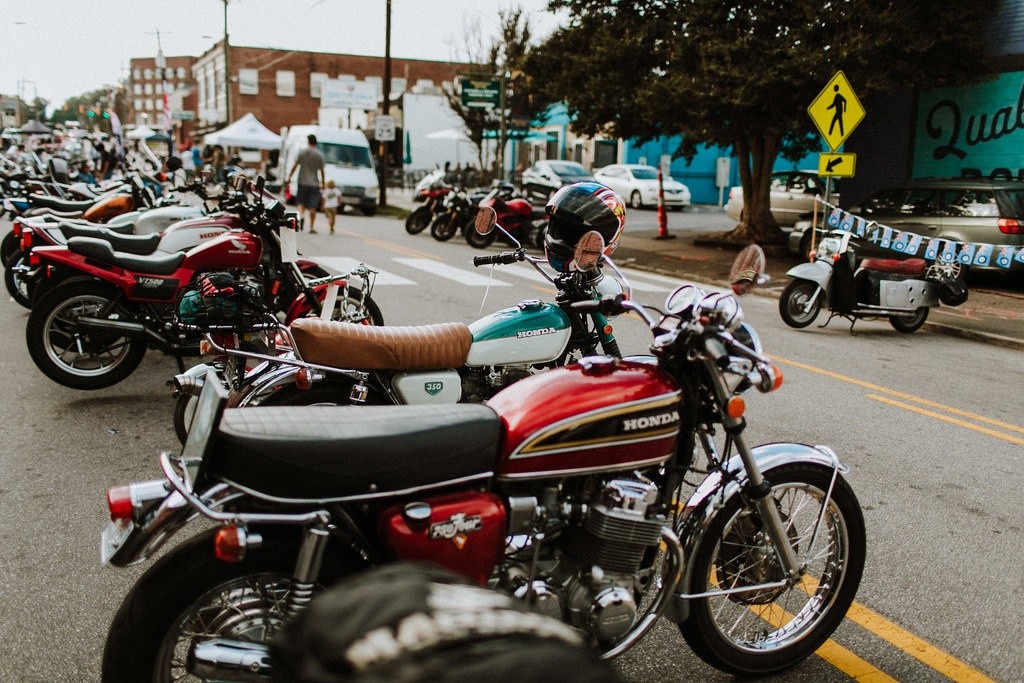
[778,220,941,336]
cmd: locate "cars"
[521,159,594,204]
[724,169,873,227]
[590,163,691,211]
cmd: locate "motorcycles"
[0,115,384,394]
[179,204,673,490]
[404,169,553,250]
[99,228,866,683]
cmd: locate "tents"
[203,112,282,152]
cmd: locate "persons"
[179,139,226,185]
[321,181,343,236]
[285,135,325,233]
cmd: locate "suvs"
[797,175,1024,284]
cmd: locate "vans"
[278,125,380,215]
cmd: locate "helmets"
[543,182,626,272]
